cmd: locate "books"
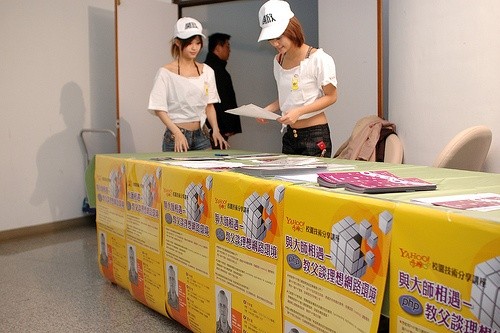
[231,163,438,195]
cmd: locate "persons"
[148,17,231,153]
[202,32,241,150]
[257,0,338,159]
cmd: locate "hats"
[258,0,295,42]
[174,16,207,40]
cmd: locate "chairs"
[336,128,403,164]
[434,126,493,172]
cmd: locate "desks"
[94,151,500,333]
